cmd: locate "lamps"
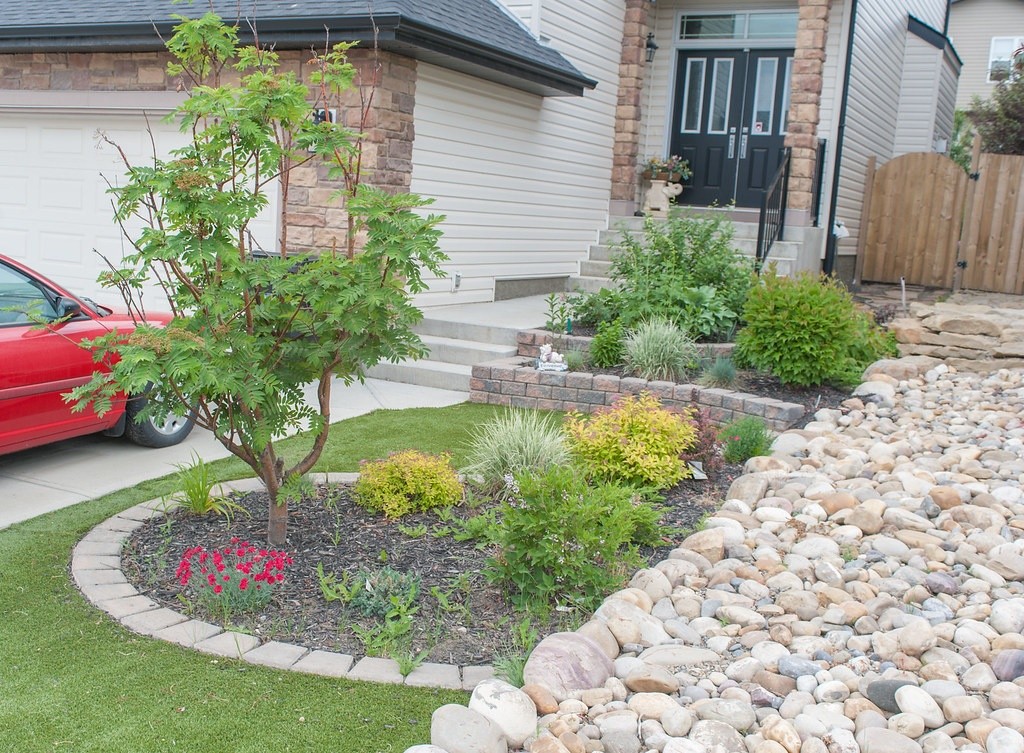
[302,109,336,153]
[646,31,660,63]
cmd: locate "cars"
[0,254,211,458]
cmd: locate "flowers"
[637,156,694,188]
